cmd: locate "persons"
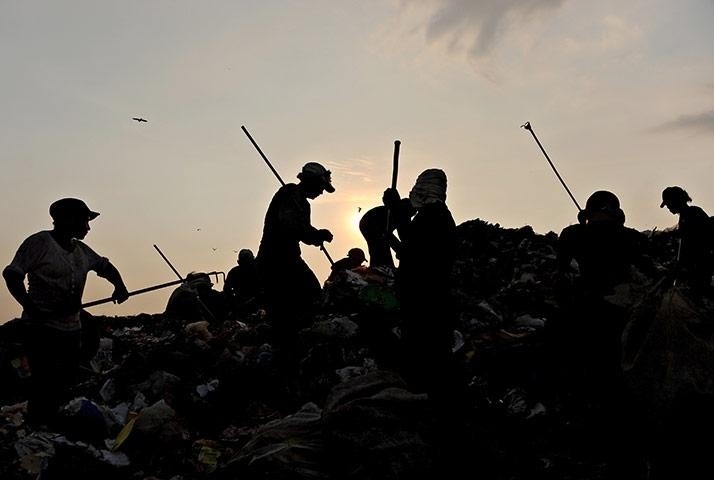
[660,185,714,305]
[224,250,265,316]
[244,162,335,349]
[1,198,131,405]
[329,247,368,282]
[547,190,659,410]
[385,168,471,402]
[362,197,416,270]
[163,275,225,339]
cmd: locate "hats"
[409,169,447,204]
[660,186,684,208]
[301,163,336,193]
[346,247,368,262]
[49,197,100,221]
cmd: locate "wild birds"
[132,117,148,123]
[358,206,362,213]
